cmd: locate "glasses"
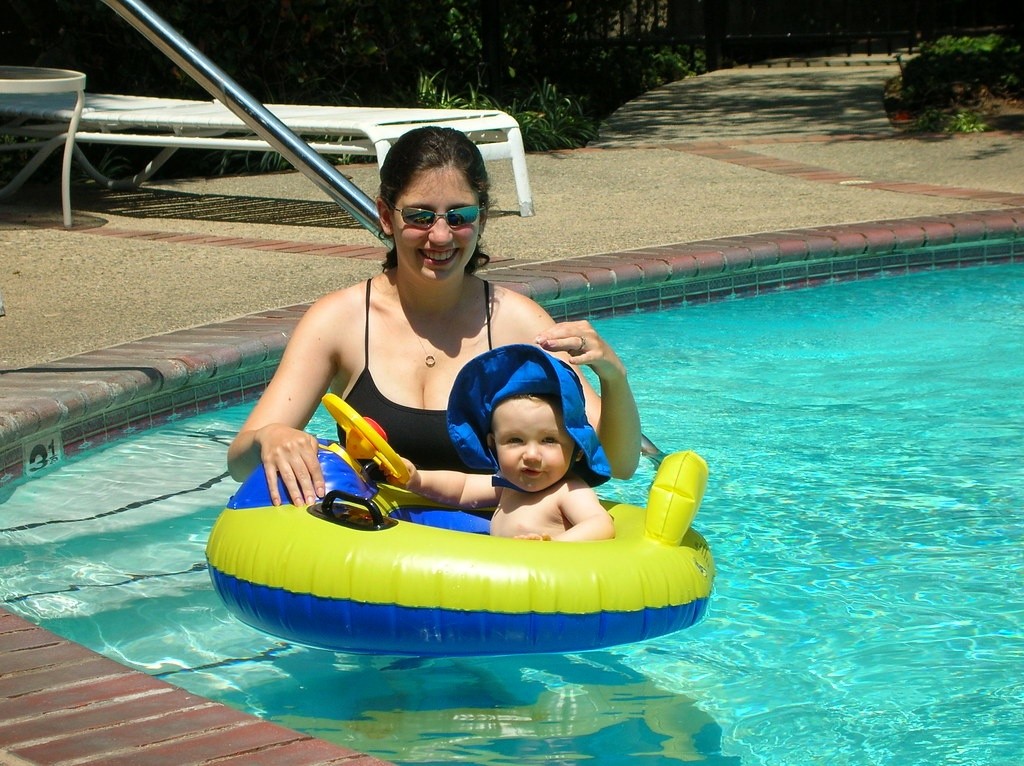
[383,196,486,229]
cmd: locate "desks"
[0,66,87,230]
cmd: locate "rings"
[576,336,585,351]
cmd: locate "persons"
[379,345,641,542]
[225,126,642,508]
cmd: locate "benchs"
[0,93,533,247]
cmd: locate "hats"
[447,342,612,491]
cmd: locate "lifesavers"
[203,434,714,653]
